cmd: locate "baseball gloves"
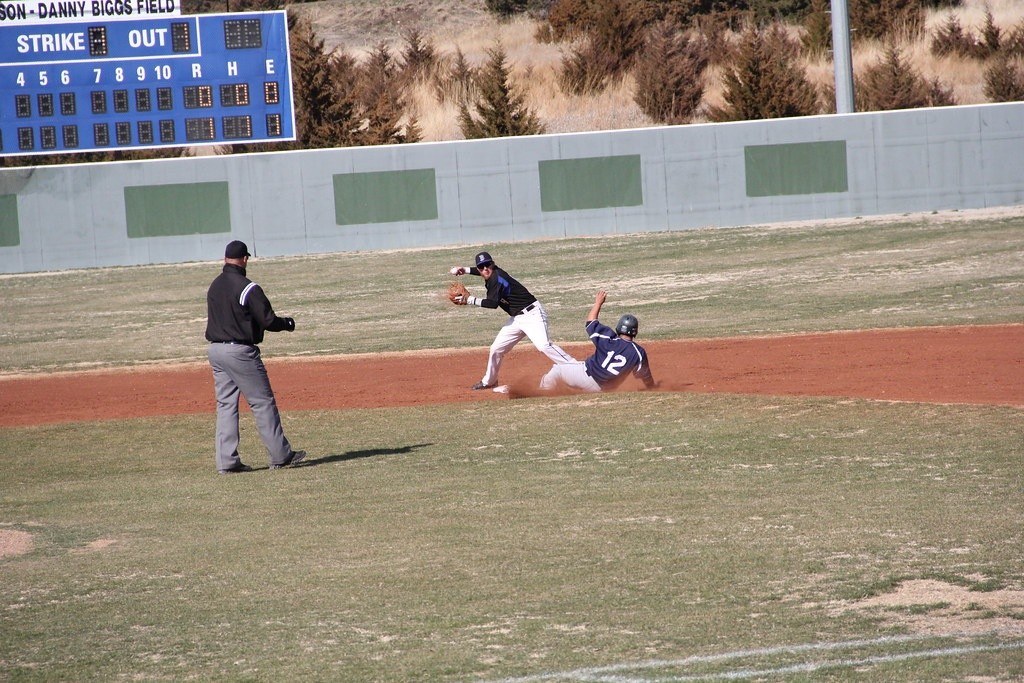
[448,284,470,305]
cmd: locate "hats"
[475,251,492,265]
[224,241,251,258]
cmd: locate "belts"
[586,370,604,389]
[211,340,240,345]
[514,305,535,316]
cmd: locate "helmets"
[615,315,638,338]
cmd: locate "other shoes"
[218,462,252,474]
[269,450,305,470]
[471,381,498,389]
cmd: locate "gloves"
[284,317,295,332]
[450,266,465,277]
[456,293,469,305]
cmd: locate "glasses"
[477,262,491,270]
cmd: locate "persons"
[538,289,656,393]
[450,252,579,390]
[205,240,307,473]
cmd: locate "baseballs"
[451,268,457,274]
